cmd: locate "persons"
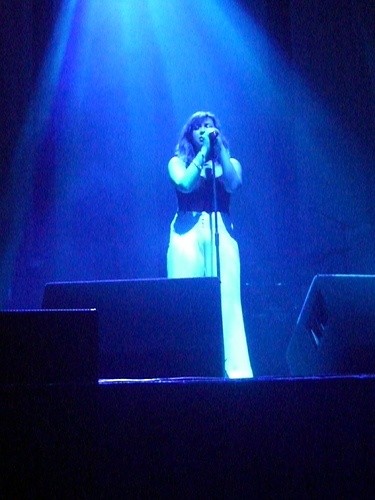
[165,110,255,379]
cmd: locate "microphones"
[209,131,219,142]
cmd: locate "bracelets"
[192,159,202,171]
[200,151,206,162]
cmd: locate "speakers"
[285,273,374,379]
[0,307,104,384]
[40,277,223,378]
